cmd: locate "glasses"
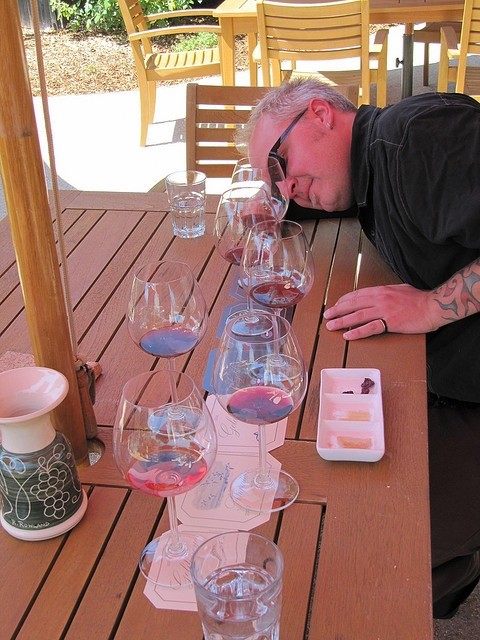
[268,108,307,206]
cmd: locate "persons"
[237,77,480,620]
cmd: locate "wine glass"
[240,219,314,381]
[215,313,308,513]
[230,157,289,288]
[213,187,279,337]
[111,370,218,588]
[127,260,208,437]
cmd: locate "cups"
[165,171,207,239]
[190,531,285,640]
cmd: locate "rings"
[379,319,388,333]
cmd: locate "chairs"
[437,0,480,106]
[147,83,281,195]
[253,0,389,113]
[412,19,461,85]
[118,0,222,146]
